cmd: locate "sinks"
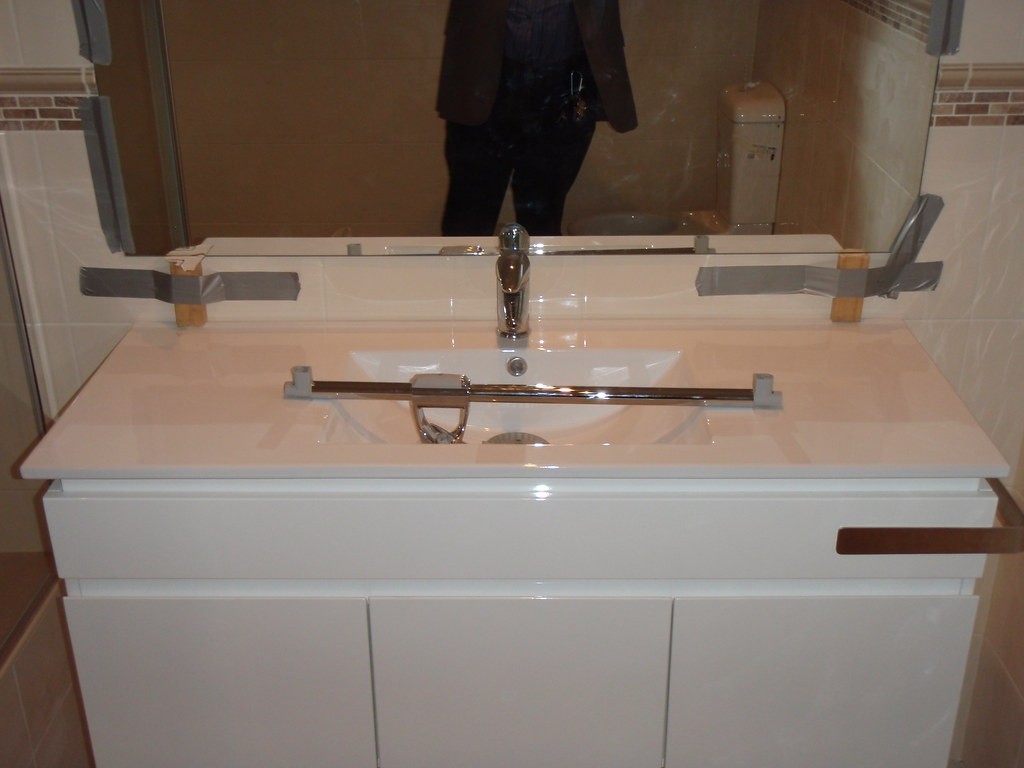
[318,343,716,451]
[383,243,654,254]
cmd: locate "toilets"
[566,78,785,238]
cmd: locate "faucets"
[499,223,530,254]
[494,252,532,340]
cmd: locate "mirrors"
[80,0,949,256]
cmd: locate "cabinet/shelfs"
[42,478,999,768]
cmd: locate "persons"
[435,0,639,236]
